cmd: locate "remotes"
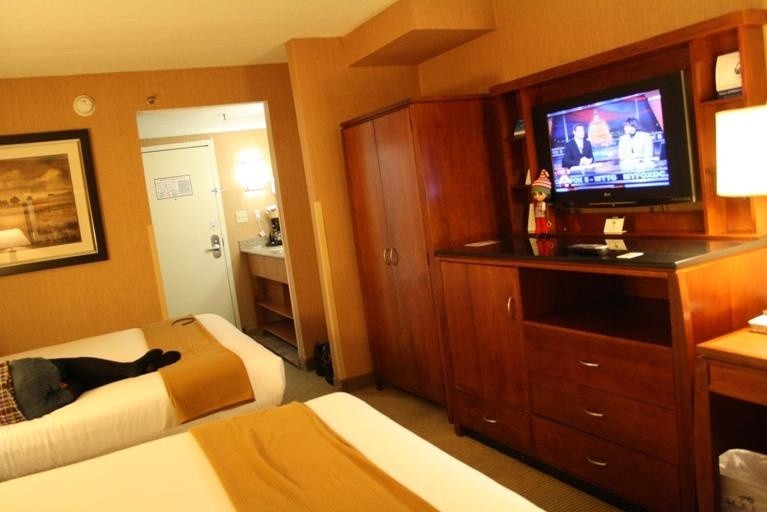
[567,243,609,256]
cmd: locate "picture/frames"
[0,128,110,277]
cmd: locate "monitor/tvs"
[532,69,697,206]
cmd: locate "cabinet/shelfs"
[432,246,763,512]
[338,94,494,409]
[238,242,296,350]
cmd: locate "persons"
[0,348,181,427]
[562,123,594,168]
[532,169,553,239]
[618,118,653,173]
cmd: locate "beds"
[0,392,546,511]
[0,313,287,476]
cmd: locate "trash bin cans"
[719,448,767,512]
